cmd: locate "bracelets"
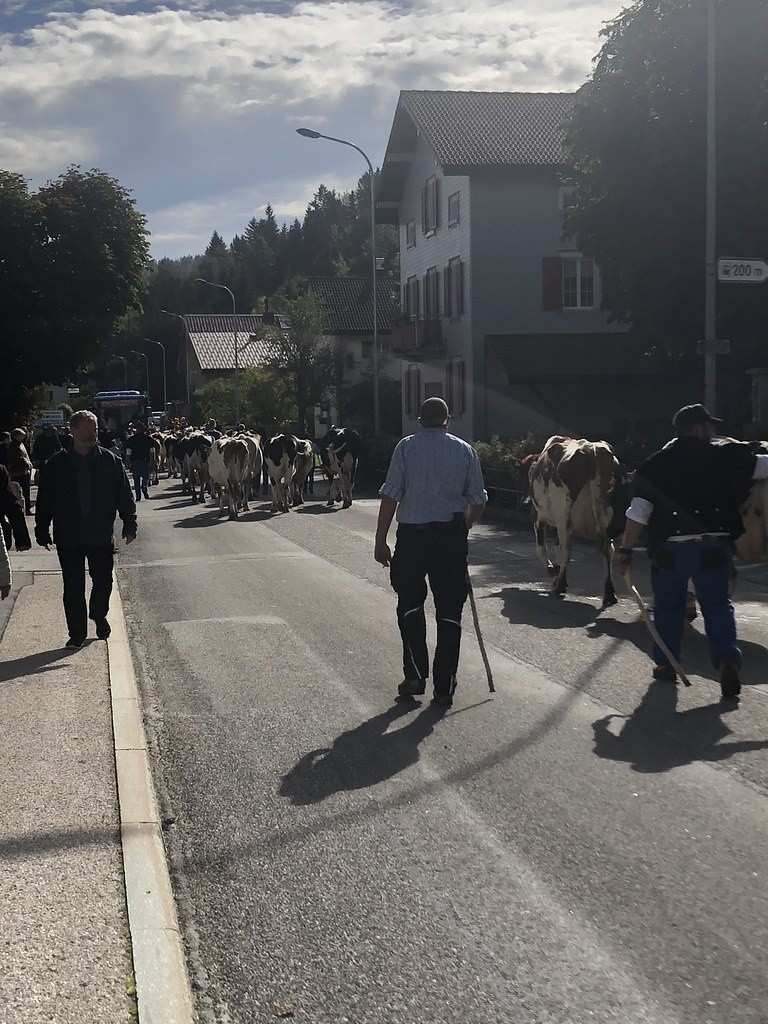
[619,544,634,555]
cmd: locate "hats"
[671,404,723,438]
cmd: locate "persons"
[164,415,245,431]
[98,419,161,501]
[0,422,35,599]
[372,394,488,705]
[29,421,74,470]
[33,410,138,649]
[618,402,768,698]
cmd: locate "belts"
[404,521,464,531]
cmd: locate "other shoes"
[142,487,151,498]
[66,635,86,649]
[720,662,741,697]
[433,686,454,706]
[135,495,141,502]
[653,661,677,680]
[398,676,426,694]
[26,509,37,515]
[93,613,110,638]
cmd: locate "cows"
[518,435,621,608]
[149,425,360,521]
[606,437,768,623]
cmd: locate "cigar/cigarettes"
[382,565,386,568]
